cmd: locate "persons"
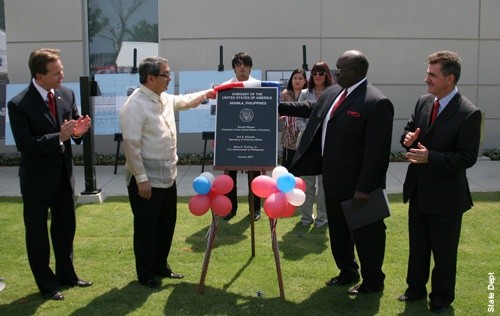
[397,51,482,313]
[119,56,216,288]
[295,62,334,228]
[278,50,394,295]
[279,69,308,169]
[7,48,94,300]
[217,52,261,220]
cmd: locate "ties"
[330,88,348,119]
[46,91,57,116]
[428,100,441,126]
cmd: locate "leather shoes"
[429,303,451,313]
[347,282,384,295]
[43,290,65,301]
[224,212,237,220]
[65,279,93,288]
[139,278,162,288]
[325,274,360,285]
[397,291,428,302]
[163,272,184,279]
[251,208,261,221]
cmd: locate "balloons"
[213,175,234,194]
[251,175,277,198]
[295,176,306,192]
[285,203,296,217]
[272,166,288,180]
[201,172,215,189]
[263,193,285,219]
[188,194,210,216]
[276,172,295,193]
[210,195,233,217]
[285,189,306,206]
[192,176,211,195]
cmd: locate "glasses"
[155,72,171,78]
[336,67,347,73]
[311,71,326,76]
[235,61,250,67]
